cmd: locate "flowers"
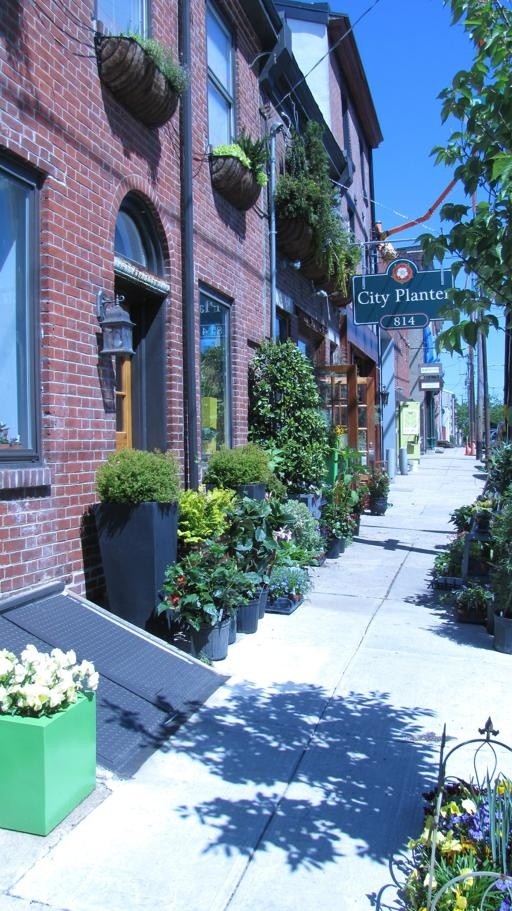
[0,644,98,716]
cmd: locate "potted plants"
[95,31,186,129]
[430,443,512,655]
[208,123,269,211]
[89,445,307,663]
[277,121,363,306]
[247,336,387,559]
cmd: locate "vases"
[0,692,101,838]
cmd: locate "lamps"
[96,291,137,357]
